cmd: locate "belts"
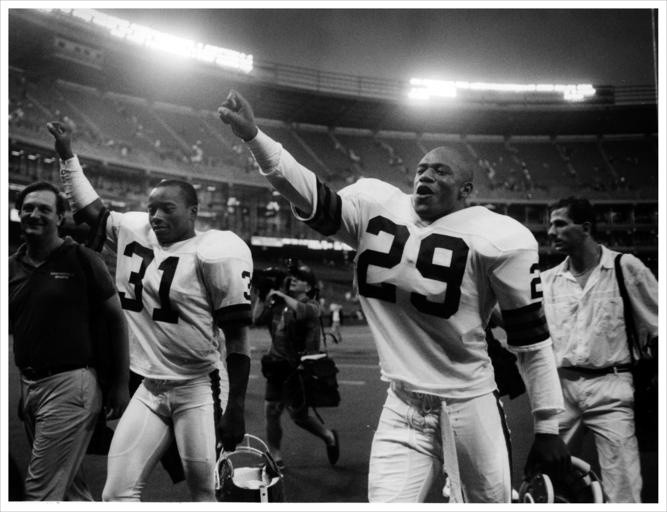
[556,363,633,380]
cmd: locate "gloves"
[216,353,251,453]
[523,434,572,479]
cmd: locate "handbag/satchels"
[633,359,656,415]
[288,352,341,408]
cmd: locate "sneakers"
[326,428,340,465]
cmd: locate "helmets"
[214,451,284,502]
[518,456,605,503]
